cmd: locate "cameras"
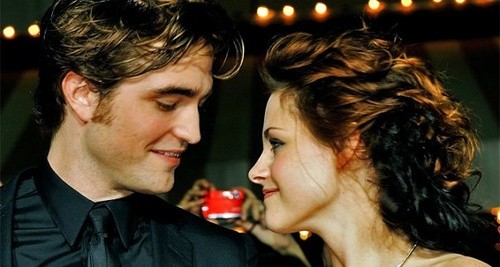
[201,189,245,219]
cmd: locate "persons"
[248,29,500,267]
[0,0,305,267]
[201,188,311,267]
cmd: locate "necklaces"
[396,241,418,267]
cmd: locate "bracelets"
[247,221,259,234]
